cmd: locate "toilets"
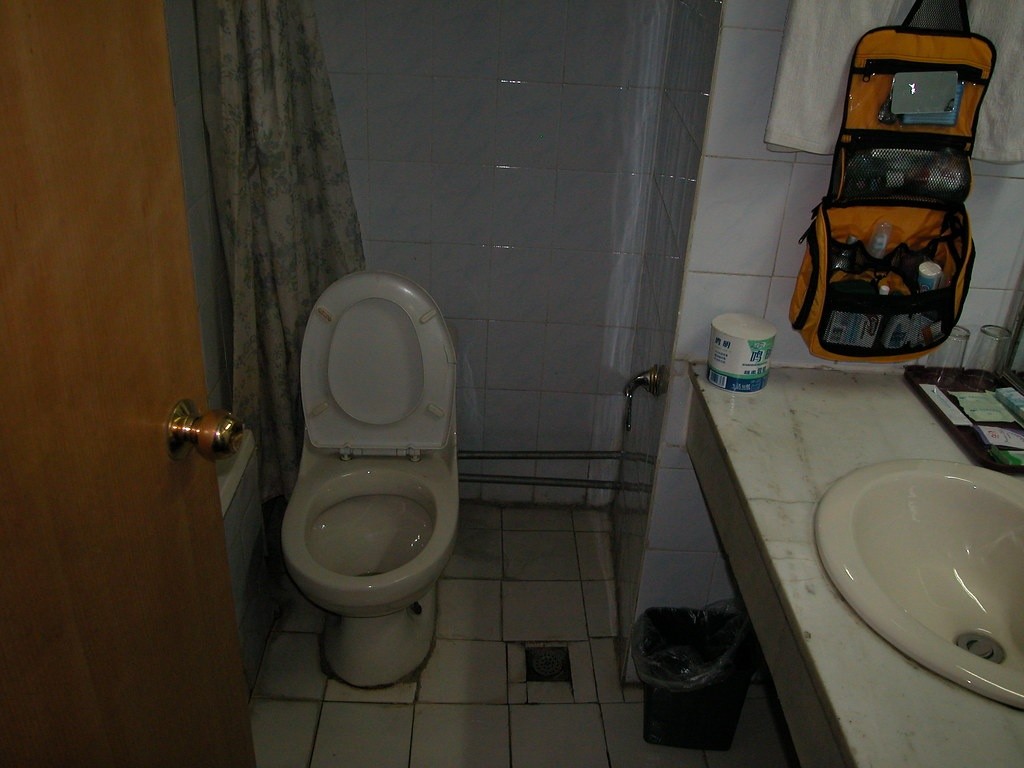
[279,270,459,689]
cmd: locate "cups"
[924,325,970,387]
[961,324,1013,389]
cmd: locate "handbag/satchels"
[788,0,998,363]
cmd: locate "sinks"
[812,458,1024,710]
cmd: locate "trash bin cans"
[642,606,754,751]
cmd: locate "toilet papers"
[706,312,777,393]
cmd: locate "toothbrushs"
[872,233,887,259]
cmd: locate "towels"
[762,1,1024,165]
